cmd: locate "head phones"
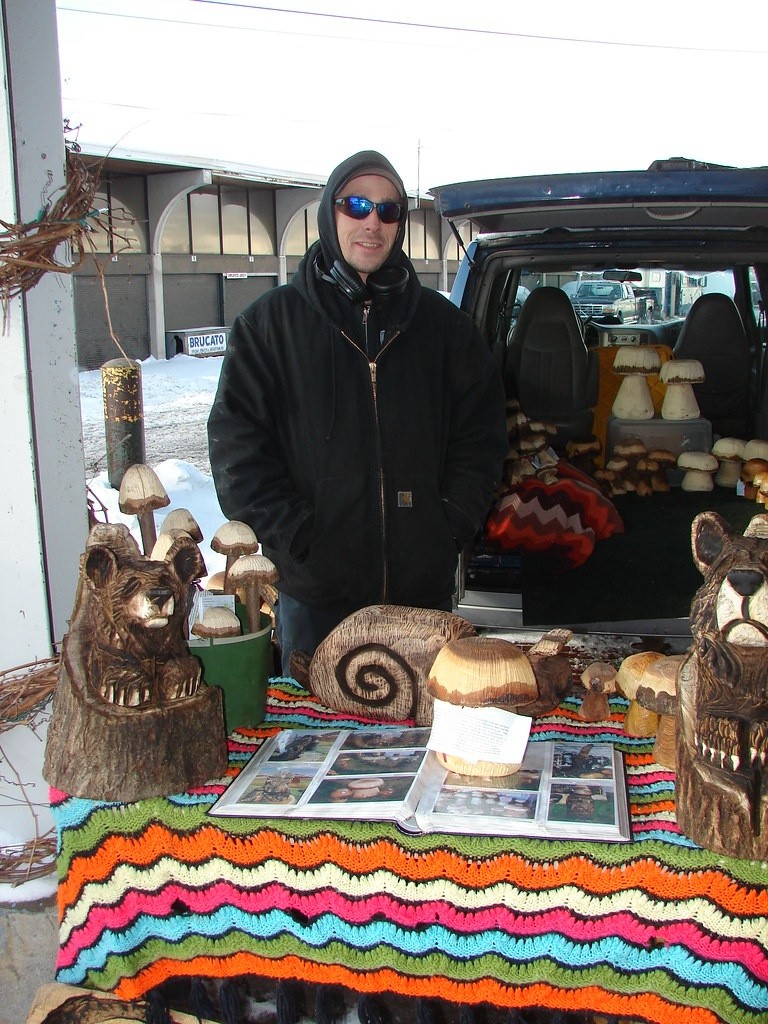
[313,253,410,305]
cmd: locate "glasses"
[334,196,402,224]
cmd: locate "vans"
[428,157,768,627]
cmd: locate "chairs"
[675,288,752,441]
[501,284,594,428]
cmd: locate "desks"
[50,675,768,1024]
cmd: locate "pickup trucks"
[570,282,656,325]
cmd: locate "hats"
[348,167,403,199]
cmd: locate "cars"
[512,286,531,316]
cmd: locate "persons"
[206,150,505,688]
